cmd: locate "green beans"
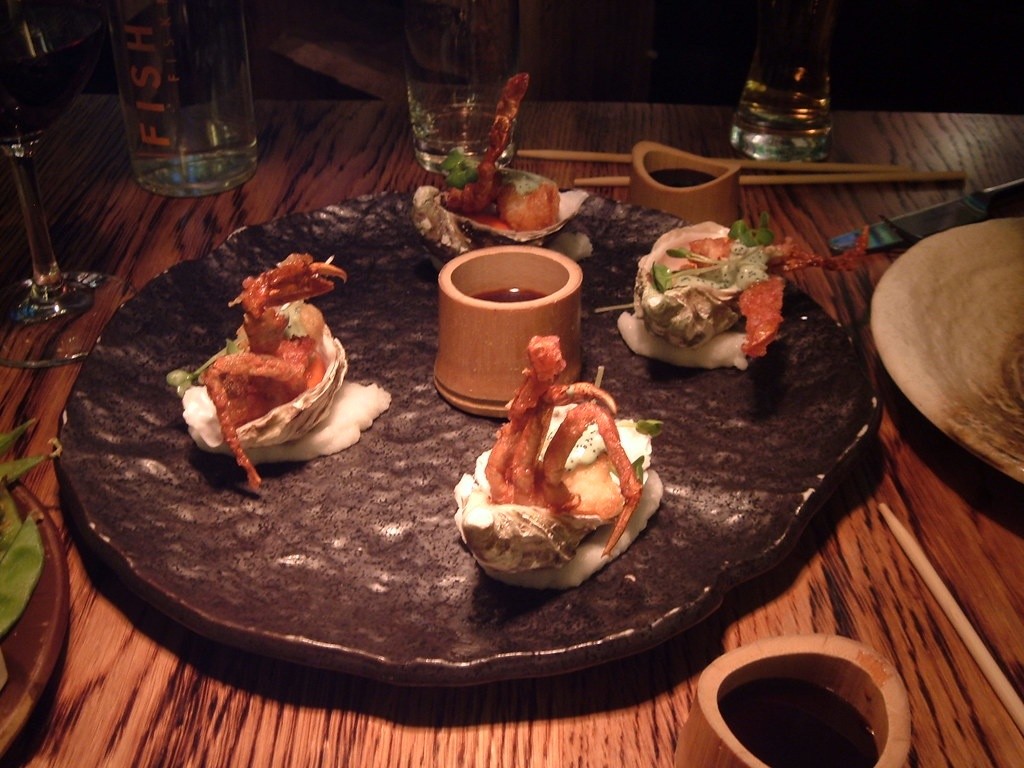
[0,416,65,646]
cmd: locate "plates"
[0,478,71,768]
[870,217,1024,488]
[51,189,884,688]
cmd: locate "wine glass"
[0,0,138,370]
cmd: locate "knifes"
[827,178,1024,255]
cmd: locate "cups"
[401,0,518,174]
[730,0,838,165]
[624,140,741,229]
[433,245,584,419]
[104,0,257,199]
[674,631,912,768]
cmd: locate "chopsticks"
[516,148,967,187]
[879,504,1024,737]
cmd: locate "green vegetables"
[165,332,247,396]
[614,416,665,484]
[650,210,775,294]
[440,148,485,190]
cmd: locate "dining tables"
[0,100,1024,768]
